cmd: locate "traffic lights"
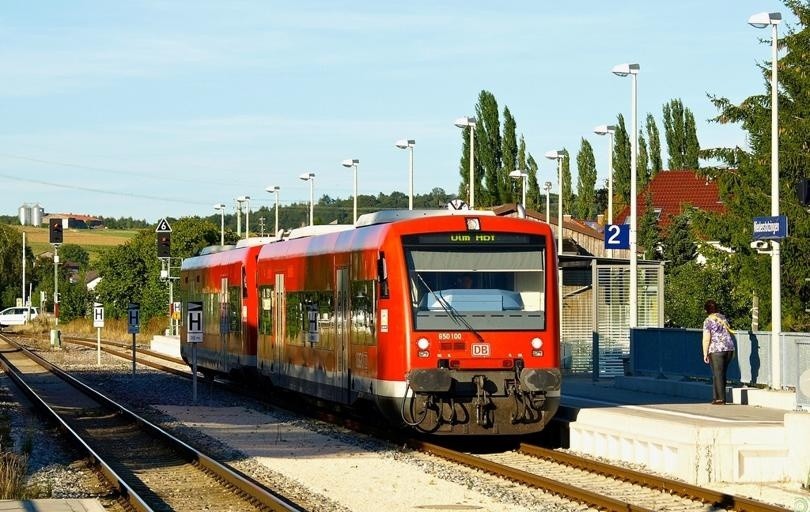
[49,216,63,245]
[157,231,171,258]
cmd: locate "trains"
[177,205,562,448]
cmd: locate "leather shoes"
[712,399,727,406]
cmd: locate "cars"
[0,306,43,328]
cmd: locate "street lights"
[454,113,480,210]
[214,189,251,246]
[511,169,532,211]
[611,61,643,329]
[596,119,618,261]
[546,147,567,257]
[397,139,417,211]
[265,184,281,237]
[299,172,316,226]
[749,7,785,391]
[343,158,362,224]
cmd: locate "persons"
[702,300,735,405]
